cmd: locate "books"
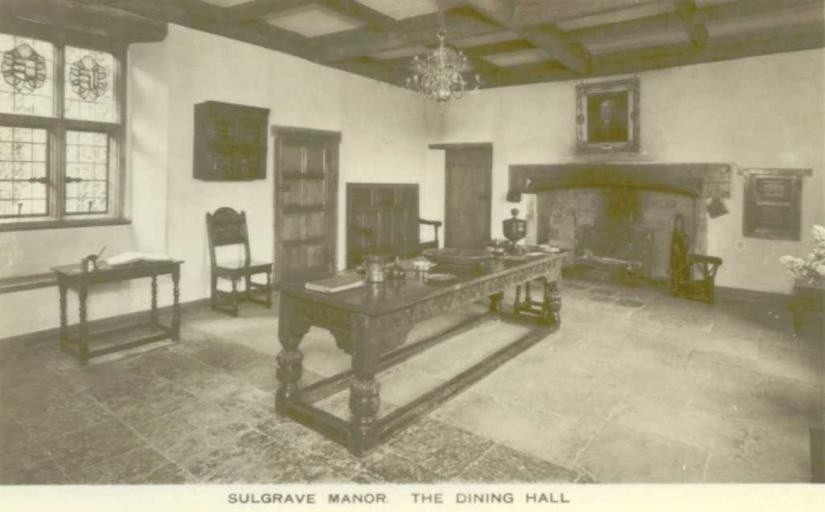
[304,273,364,294]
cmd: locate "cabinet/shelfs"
[193,101,270,180]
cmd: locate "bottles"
[388,256,406,279]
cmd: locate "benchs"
[347,183,442,268]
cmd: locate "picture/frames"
[575,78,639,154]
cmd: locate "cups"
[361,253,385,283]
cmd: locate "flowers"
[779,224,825,287]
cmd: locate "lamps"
[404,0,485,114]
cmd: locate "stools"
[689,254,723,305]
[514,277,547,319]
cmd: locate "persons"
[591,99,627,141]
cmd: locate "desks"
[791,278,825,336]
[50,258,185,365]
[274,244,570,458]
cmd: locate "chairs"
[206,207,274,316]
